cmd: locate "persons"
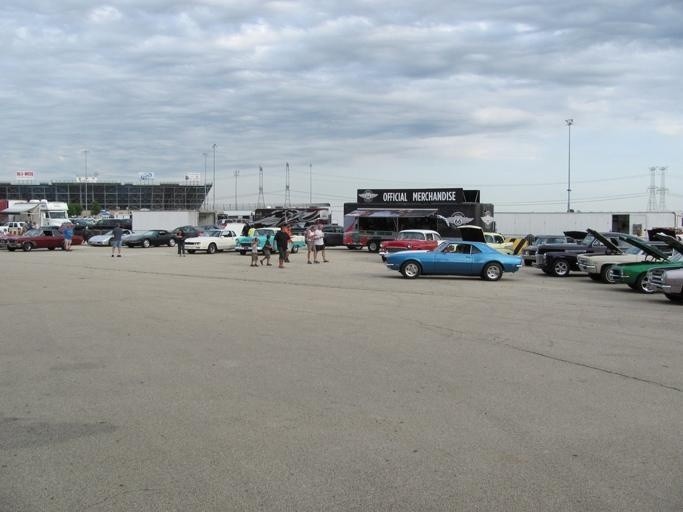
[112,223,122,257]
[242,223,250,236]
[250,238,259,267]
[273,223,294,268]
[247,225,256,236]
[177,230,186,257]
[284,224,292,263]
[260,234,272,266]
[63,222,73,251]
[305,225,320,264]
[456,246,461,251]
[455,246,465,253]
[314,224,329,263]
[22,220,37,233]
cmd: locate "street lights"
[201,153,206,210]
[564,118,573,213]
[81,149,87,211]
[211,143,216,211]
[233,168,238,211]
